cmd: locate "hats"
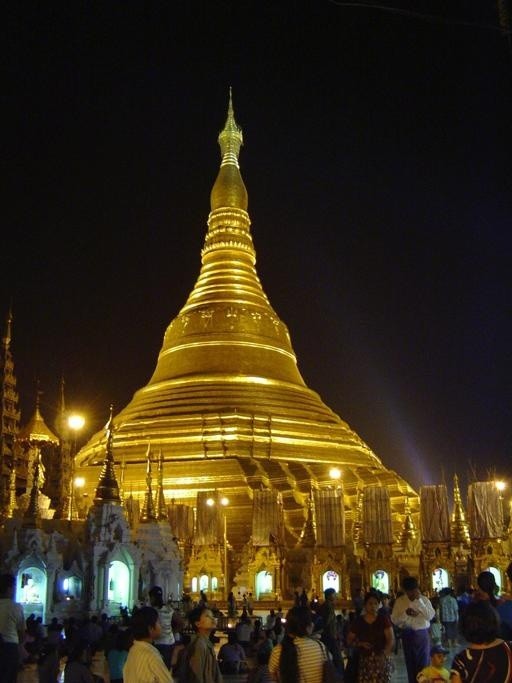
[430,644,449,657]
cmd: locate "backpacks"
[173,635,200,682]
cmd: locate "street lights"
[329,467,347,601]
[220,497,231,600]
[67,413,88,520]
[496,480,506,524]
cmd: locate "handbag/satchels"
[315,639,344,682]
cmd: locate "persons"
[1,560,511,682]
